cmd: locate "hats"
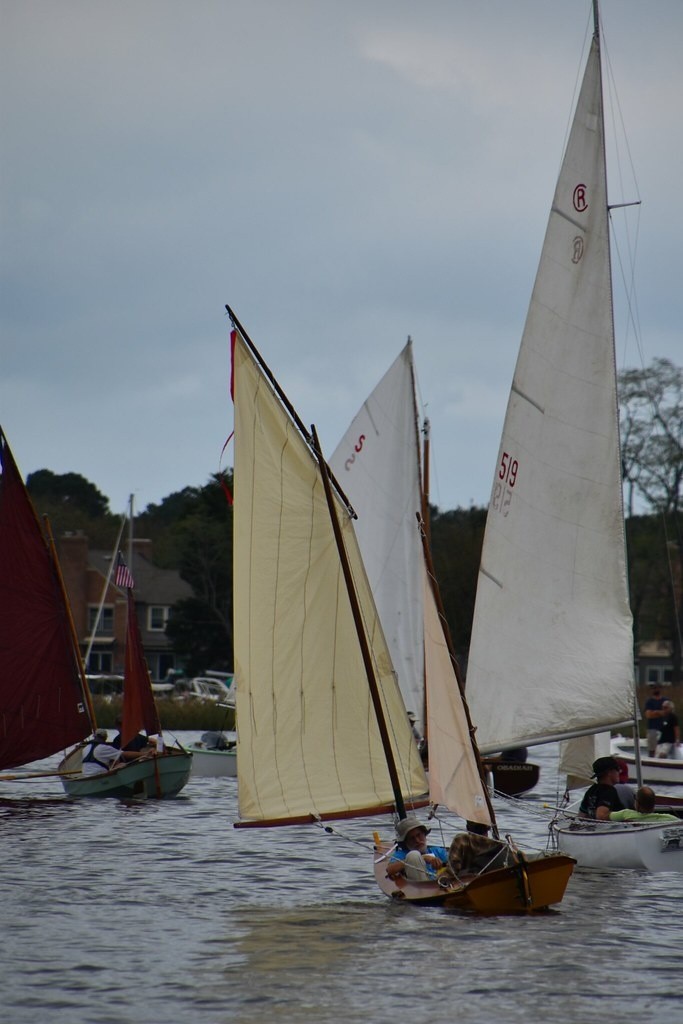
[408,714,420,721]
[392,816,431,843]
[93,728,108,738]
[613,757,628,783]
[590,757,618,779]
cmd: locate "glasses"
[616,767,619,772]
[633,794,637,800]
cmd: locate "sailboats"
[0,426,192,799]
[450,0,682,873]
[223,303,579,918]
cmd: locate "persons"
[643,682,680,759]
[386,817,448,880]
[466,819,489,836]
[82,712,165,777]
[579,757,682,822]
[407,711,421,744]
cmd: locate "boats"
[608,734,683,785]
[183,742,242,778]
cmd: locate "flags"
[115,553,134,588]
